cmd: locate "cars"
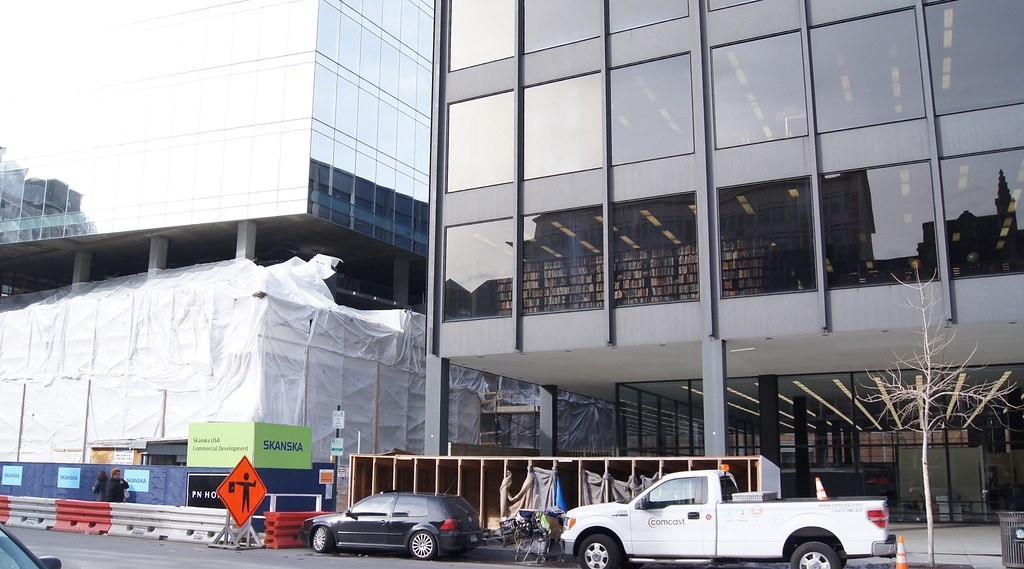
[0,525,63,569]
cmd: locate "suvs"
[300,489,482,561]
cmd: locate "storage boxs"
[731,491,778,502]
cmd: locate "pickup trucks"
[559,470,898,569]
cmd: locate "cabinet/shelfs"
[492,234,765,315]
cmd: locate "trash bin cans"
[996,512,1024,568]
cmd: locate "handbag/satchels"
[120,479,130,498]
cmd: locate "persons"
[92,471,108,502]
[105,468,129,502]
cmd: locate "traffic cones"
[895,536,909,569]
[815,476,830,501]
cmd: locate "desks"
[937,502,975,521]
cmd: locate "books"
[497,238,762,316]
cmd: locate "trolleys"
[513,519,563,564]
